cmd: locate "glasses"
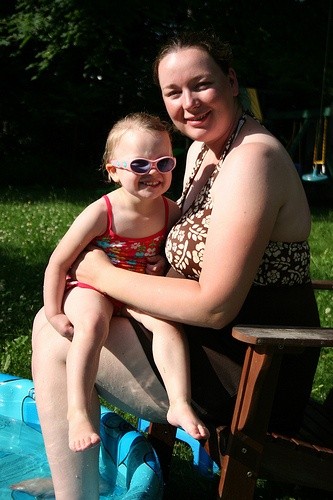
[110,155,177,176]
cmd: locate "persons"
[43,113,210,452]
[12,32,322,500]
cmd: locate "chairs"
[201,279,333,499]
